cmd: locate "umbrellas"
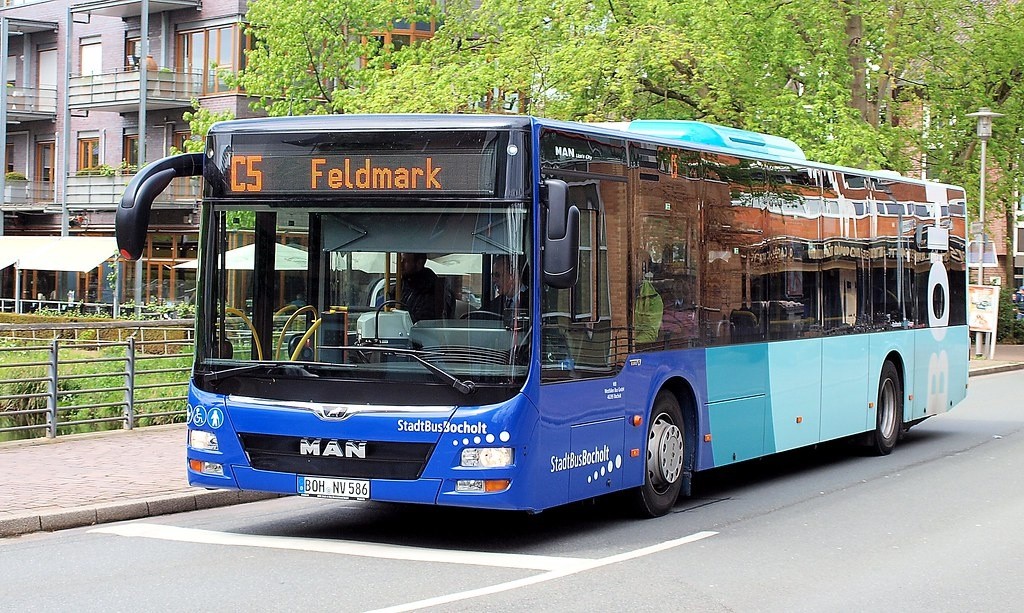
[172,241,482,275]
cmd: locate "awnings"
[0,236,62,271]
[15,236,119,272]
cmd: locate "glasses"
[492,272,500,278]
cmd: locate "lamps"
[69,219,82,228]
[127,54,139,70]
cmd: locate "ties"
[506,299,513,308]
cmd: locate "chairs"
[730,311,765,343]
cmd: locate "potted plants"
[158,68,173,99]
[115,158,137,177]
[6,84,15,109]
[3,172,31,203]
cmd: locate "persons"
[388,251,445,322]
[794,272,801,291]
[1012,285,1024,319]
[633,247,663,343]
[289,293,307,314]
[467,255,529,320]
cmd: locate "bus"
[114,114,970,521]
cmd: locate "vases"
[14,96,25,110]
[138,55,158,96]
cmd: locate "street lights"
[964,104,1008,356]
